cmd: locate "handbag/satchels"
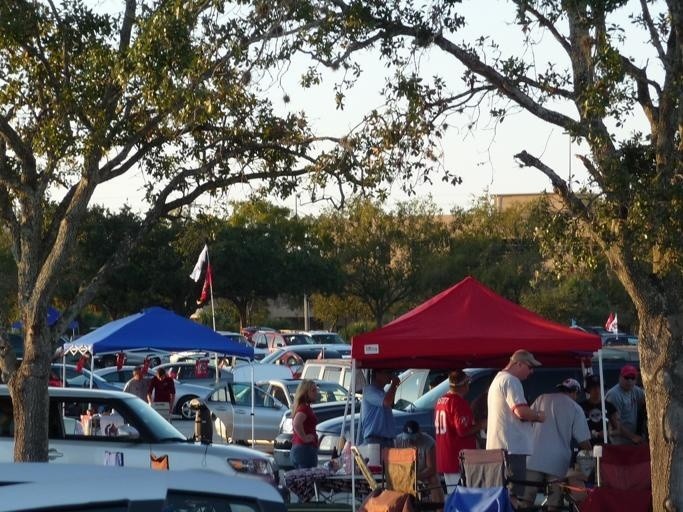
[357,488,417,512]
[443,484,509,512]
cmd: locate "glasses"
[520,361,532,370]
[376,368,391,374]
[620,374,636,381]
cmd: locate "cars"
[206,376,363,446]
[1,347,254,394]
[229,343,352,383]
[143,364,236,421]
[302,323,644,468]
[1,387,281,485]
[1,462,290,510]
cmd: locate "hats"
[619,365,640,375]
[509,349,541,367]
[553,379,580,393]
[401,420,418,441]
[581,374,605,394]
[447,372,472,387]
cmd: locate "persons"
[146,368,176,424]
[84,377,98,388]
[123,367,148,403]
[49,371,63,387]
[567,376,621,487]
[104,423,111,437]
[486,349,544,509]
[360,368,400,448]
[80,409,93,436]
[292,378,320,468]
[435,369,480,495]
[517,378,592,508]
[218,353,229,371]
[605,366,643,465]
[394,420,445,508]
[168,366,176,378]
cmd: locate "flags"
[189,243,212,305]
[606,310,618,339]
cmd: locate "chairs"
[356,442,654,512]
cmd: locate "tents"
[348,277,607,512]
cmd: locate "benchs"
[277,471,424,512]
